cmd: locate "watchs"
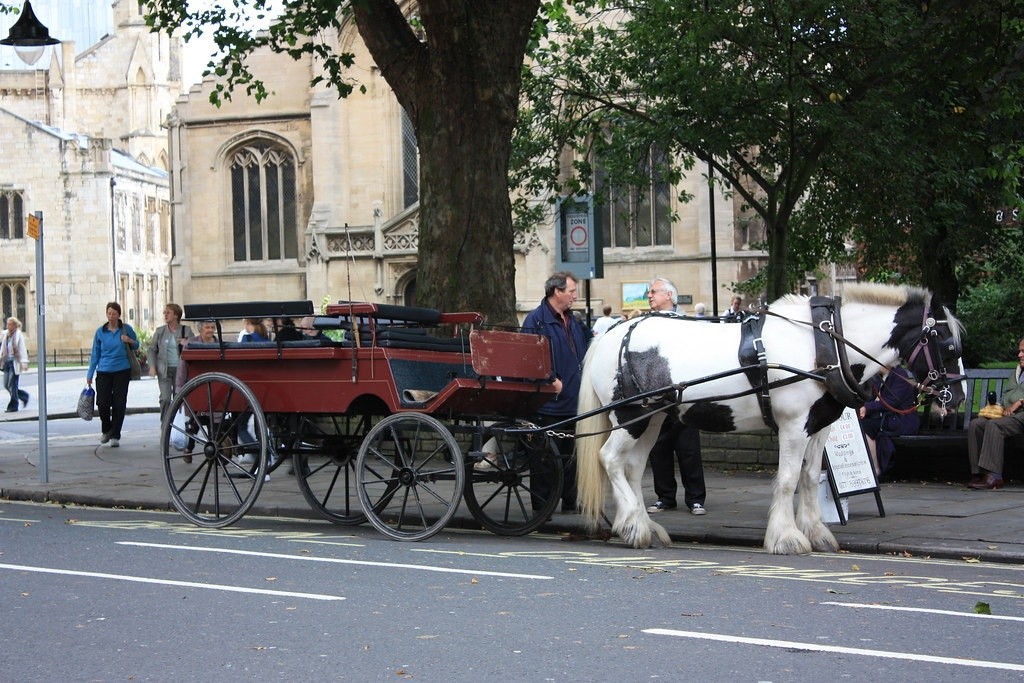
[1019,399,1024,406]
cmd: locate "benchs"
[890,368,1024,447]
[177,300,486,415]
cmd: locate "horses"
[576,281,967,556]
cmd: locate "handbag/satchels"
[77,383,95,421]
[178,325,190,357]
[168,404,189,452]
[122,323,142,381]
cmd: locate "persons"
[0,316,30,412]
[147,304,196,445]
[473,321,517,472]
[177,320,239,463]
[87,302,139,447]
[593,305,618,335]
[237,318,333,482]
[967,338,1024,490]
[691,302,706,318]
[720,296,746,323]
[521,271,596,520]
[860,360,919,481]
[648,279,708,516]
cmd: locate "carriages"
[158,257,971,557]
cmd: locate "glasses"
[163,310,175,315]
[645,289,667,295]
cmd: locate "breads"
[979,405,1003,418]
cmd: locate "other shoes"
[23,395,29,408]
[647,500,678,512]
[183,448,192,463]
[878,472,887,483]
[532,509,552,522]
[101,430,112,443]
[254,467,259,474]
[265,475,270,482]
[966,473,1004,489]
[562,504,582,514]
[691,503,706,515]
[4,409,18,413]
[289,465,310,475]
[110,438,119,447]
